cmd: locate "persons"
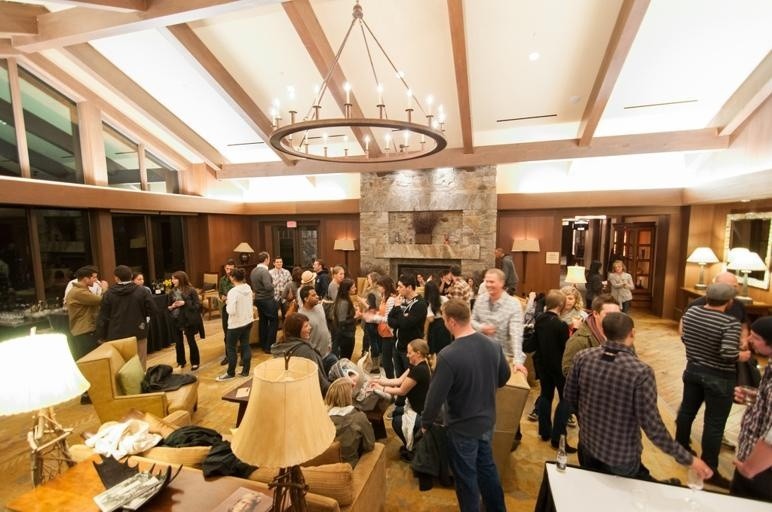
[680,271,751,451]
[495,248,520,297]
[607,259,634,312]
[420,297,510,511]
[563,314,714,482]
[471,268,528,452]
[324,377,376,470]
[585,259,604,310]
[729,316,772,504]
[368,338,431,463]
[674,281,752,490]
[522,284,635,454]
[271,312,331,399]
[63,252,205,404]
[215,251,480,381]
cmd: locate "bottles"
[557,434,568,472]
[152,279,165,295]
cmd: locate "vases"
[165,288,172,294]
[154,289,162,294]
[414,232,433,244]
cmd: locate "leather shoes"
[220,359,228,364]
[191,365,199,371]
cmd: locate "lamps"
[231,355,336,512]
[334,238,356,275]
[511,238,540,284]
[0,333,90,487]
[233,243,255,264]
[270,0,448,165]
[564,265,587,288]
[686,247,767,304]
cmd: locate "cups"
[0,296,68,325]
[739,384,759,406]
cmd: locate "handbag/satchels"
[522,324,536,354]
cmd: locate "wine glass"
[685,467,705,508]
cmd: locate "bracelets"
[383,386,385,392]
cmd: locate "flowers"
[164,278,173,288]
[151,279,164,291]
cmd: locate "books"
[637,248,650,288]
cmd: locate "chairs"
[75,335,199,423]
[192,272,219,309]
[491,358,530,488]
[232,277,369,351]
[201,289,220,320]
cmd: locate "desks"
[4,453,306,512]
[535,458,772,512]
[680,284,772,353]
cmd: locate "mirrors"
[722,210,772,290]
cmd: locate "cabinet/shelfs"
[235,263,256,289]
[611,222,656,307]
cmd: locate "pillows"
[138,446,211,466]
[116,354,146,395]
[301,441,340,467]
[247,463,356,505]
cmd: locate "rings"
[704,467,708,472]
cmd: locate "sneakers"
[567,414,577,425]
[704,470,732,489]
[722,435,736,450]
[528,409,538,419]
[217,373,232,382]
[553,445,577,453]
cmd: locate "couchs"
[69,410,387,512]
